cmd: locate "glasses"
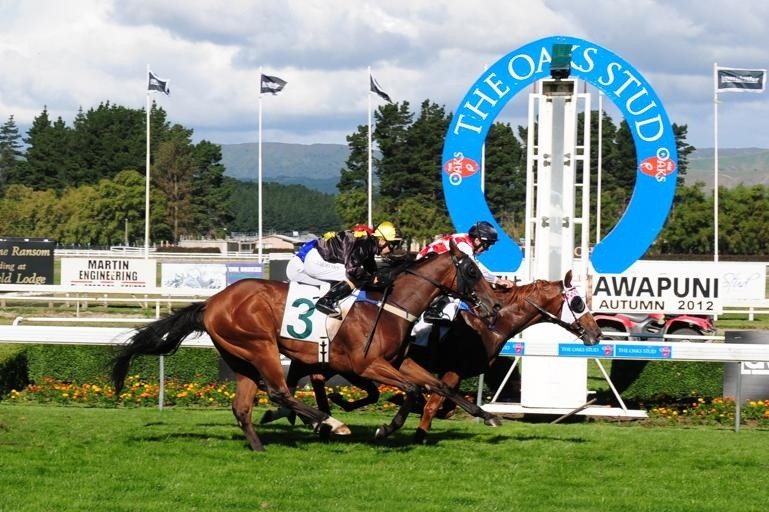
[387,242,396,249]
[482,241,490,249]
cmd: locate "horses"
[98,238,503,452]
[260,358,474,429]
[311,270,602,445]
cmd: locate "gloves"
[374,273,393,290]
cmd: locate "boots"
[315,280,352,321]
[424,296,449,320]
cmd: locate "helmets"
[370,220,404,243]
[469,221,499,245]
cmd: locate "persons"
[304,220,403,320]
[286,231,336,297]
[415,222,515,288]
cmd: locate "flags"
[148,71,170,96]
[371,75,393,103]
[716,67,766,93]
[260,74,286,94]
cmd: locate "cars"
[591,313,716,342]
